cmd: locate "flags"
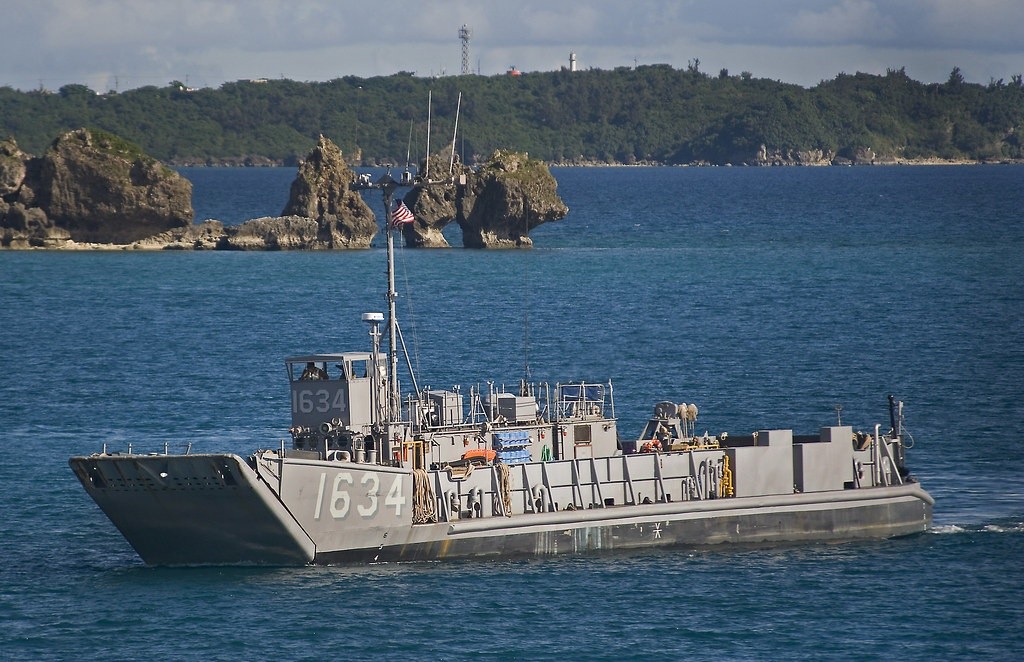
[390,198,414,228]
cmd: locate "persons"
[300,362,330,381]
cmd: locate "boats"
[66,197,934,567]
[350,174,380,190]
